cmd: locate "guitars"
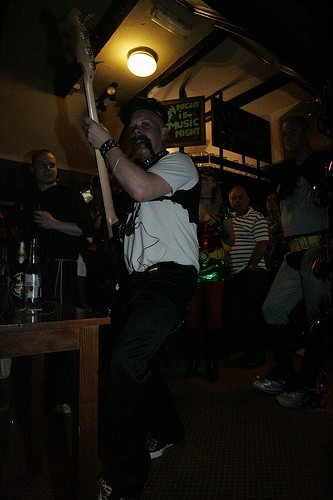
[71,13,127,307]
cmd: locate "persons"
[5,95,333,500]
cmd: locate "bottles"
[12,242,25,302]
[25,240,42,305]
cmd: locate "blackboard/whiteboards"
[159,95,206,147]
[210,97,272,164]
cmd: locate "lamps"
[107,87,116,101]
[127,47,158,78]
[97,100,107,112]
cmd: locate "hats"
[117,98,168,127]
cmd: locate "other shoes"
[178,353,200,379]
[148,435,175,460]
[52,403,72,425]
[97,468,131,500]
[206,360,219,382]
[250,375,288,394]
[275,387,309,409]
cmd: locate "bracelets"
[113,153,129,173]
[98,139,121,158]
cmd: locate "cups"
[32,204,45,211]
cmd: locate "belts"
[287,235,323,253]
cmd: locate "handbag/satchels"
[196,250,226,283]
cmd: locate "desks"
[0,301,110,500]
